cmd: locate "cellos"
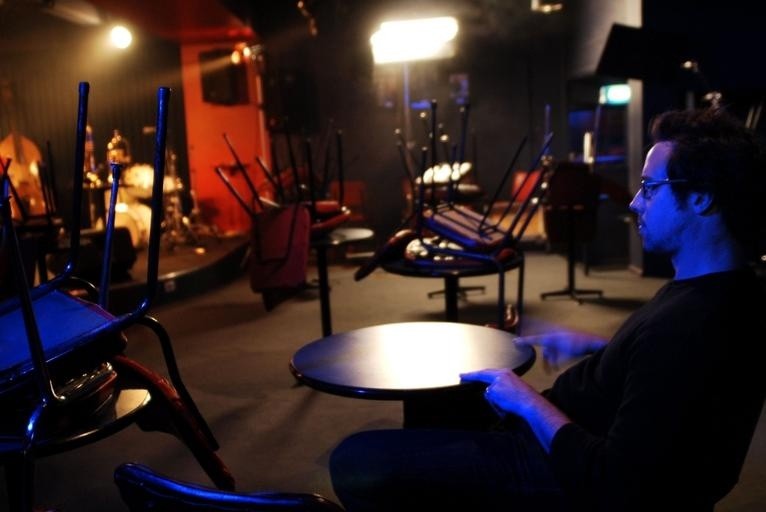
[0,79,65,242]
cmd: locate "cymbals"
[83,181,132,189]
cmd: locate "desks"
[292,321,535,511]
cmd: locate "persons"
[329,108,766,512]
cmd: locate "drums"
[96,203,152,249]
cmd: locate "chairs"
[115,464,337,511]
[207,96,604,334]
[1,78,240,489]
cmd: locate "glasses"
[640,178,689,200]
